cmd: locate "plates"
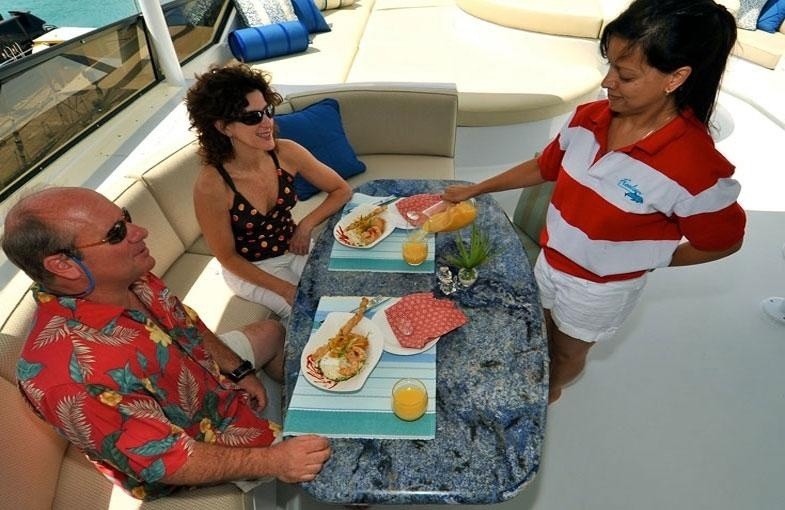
[299,310,385,393]
[332,202,396,249]
[388,197,419,230]
[370,304,440,356]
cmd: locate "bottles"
[420,195,478,235]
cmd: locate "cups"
[402,239,429,267]
[390,377,429,422]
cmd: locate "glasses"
[231,103,274,125]
[71,207,132,251]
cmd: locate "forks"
[348,294,384,314]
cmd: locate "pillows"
[268,97,366,202]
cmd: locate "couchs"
[0,82,459,508]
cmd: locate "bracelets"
[228,359,258,383]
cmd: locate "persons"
[0,185,332,502]
[435,0,749,406]
[184,61,353,322]
